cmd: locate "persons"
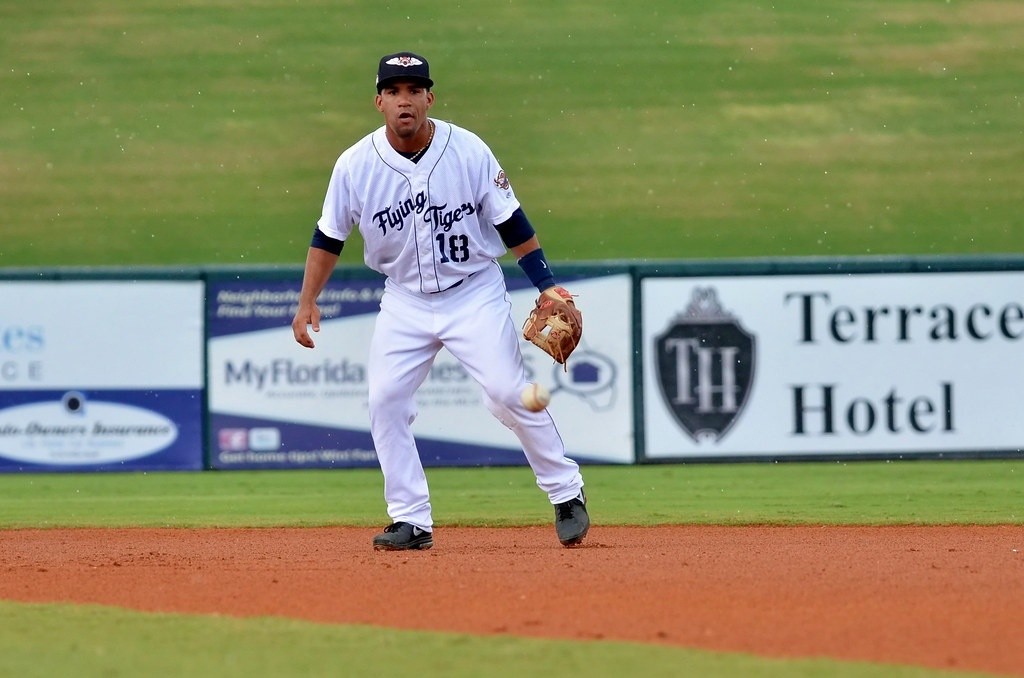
[290,52,592,554]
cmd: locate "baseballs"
[522,384,549,411]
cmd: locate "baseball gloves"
[522,286,582,372]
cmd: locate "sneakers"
[554,491,591,545]
[371,523,434,552]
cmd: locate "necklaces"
[384,121,433,161]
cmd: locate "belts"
[428,269,477,294]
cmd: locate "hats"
[375,52,435,93]
